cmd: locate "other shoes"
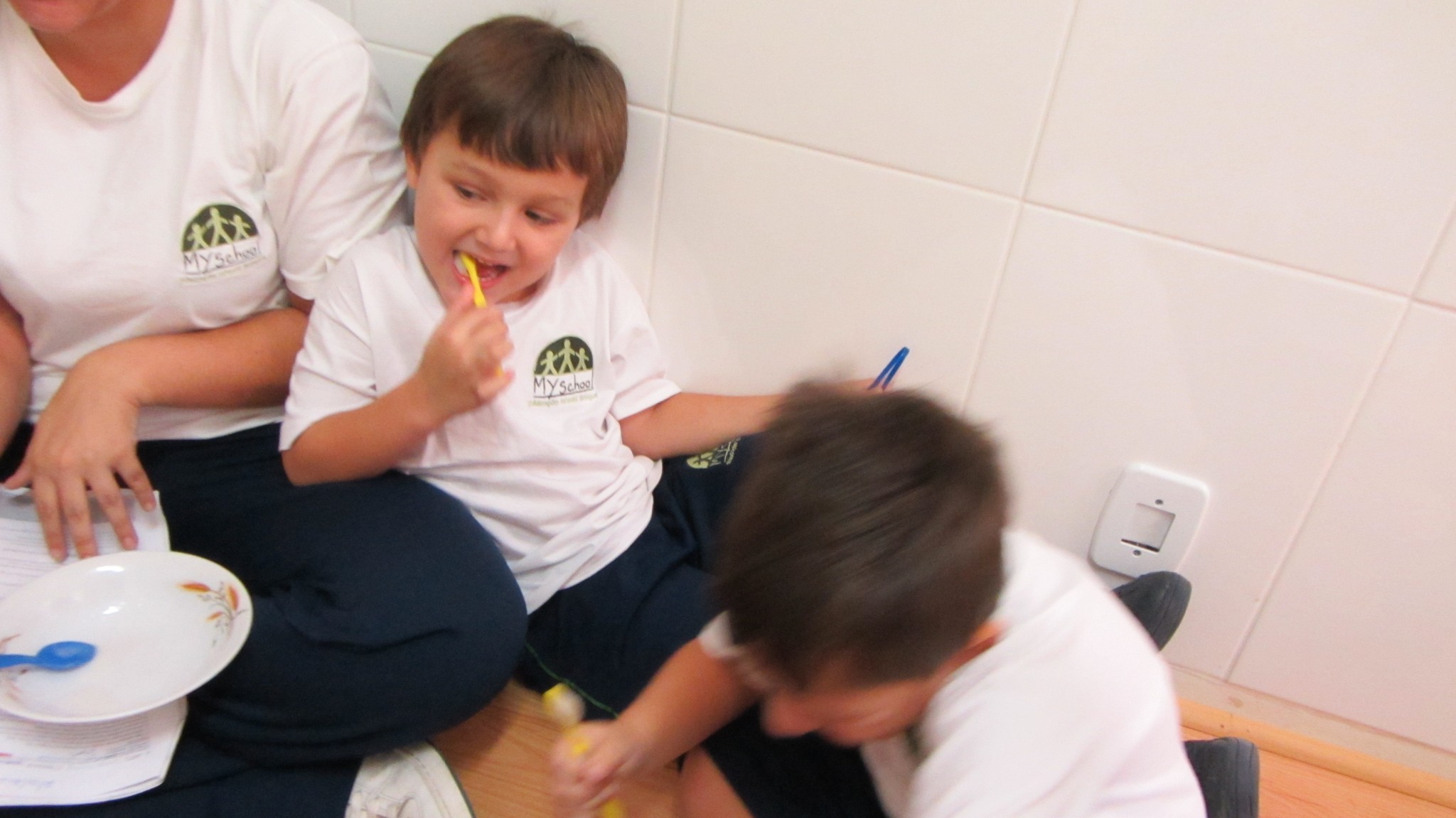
[1182,736,1259,818]
[1112,571,1191,652]
[345,738,477,818]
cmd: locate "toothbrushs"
[545,682,626,816]
[454,250,505,378]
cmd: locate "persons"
[541,381,1210,816]
[1,0,527,818]
[274,11,1266,818]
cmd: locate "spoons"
[0,642,97,670]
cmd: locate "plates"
[0,548,253,723]
